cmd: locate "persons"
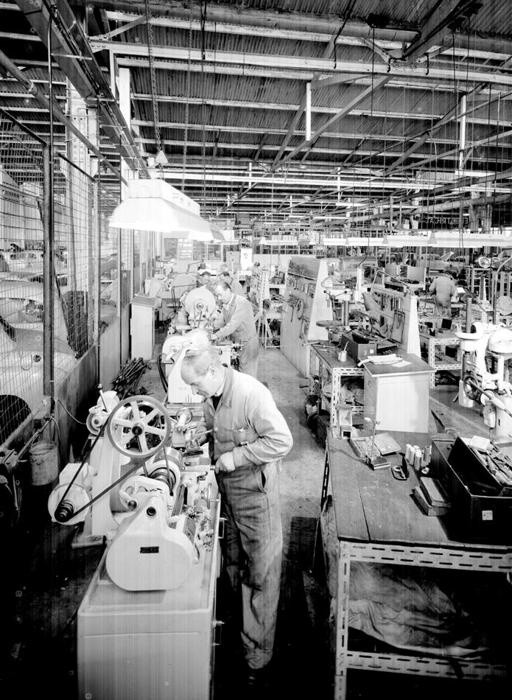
[9,243,23,260]
[427,271,468,310]
[470,251,512,272]
[177,345,294,700]
[161,262,259,403]
[406,253,415,265]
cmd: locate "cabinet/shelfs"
[246,275,467,435]
[317,429,510,699]
[130,294,156,360]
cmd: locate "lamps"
[192,101,226,244]
[110,0,212,241]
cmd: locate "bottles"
[404,443,431,471]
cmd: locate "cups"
[338,350,347,362]
